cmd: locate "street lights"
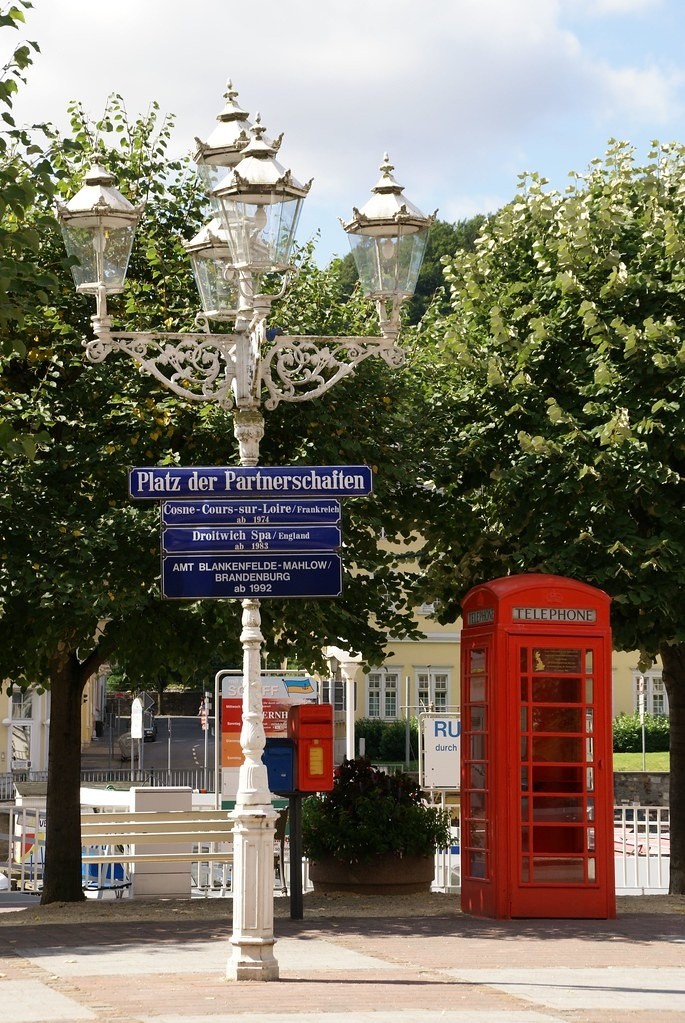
[331,636,364,760]
[53,80,440,980]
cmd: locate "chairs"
[272,805,290,896]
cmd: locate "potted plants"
[300,753,451,895]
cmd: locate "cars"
[143,712,157,741]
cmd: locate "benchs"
[79,810,236,899]
